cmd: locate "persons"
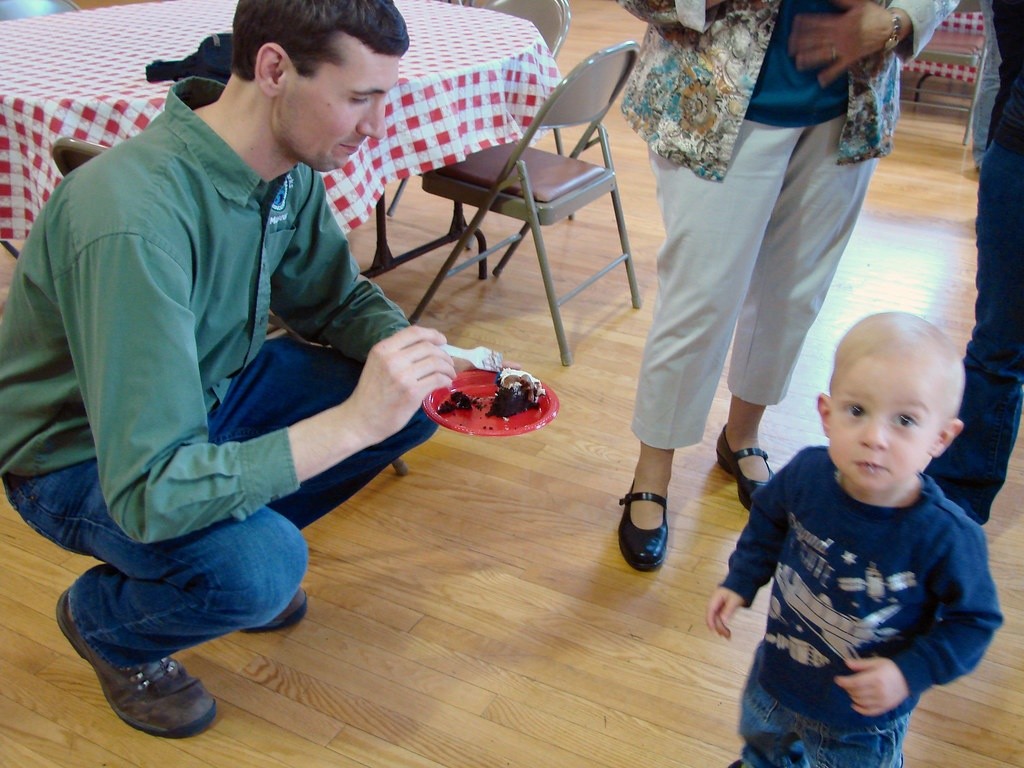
[615,0,961,566]
[702,312,1004,768]
[1,0,520,741]
[924,1,1024,529]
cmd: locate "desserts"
[439,368,545,419]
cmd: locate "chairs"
[384,0,571,216]
[407,39,642,365]
[898,0,987,147]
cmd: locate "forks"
[440,343,504,373]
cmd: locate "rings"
[832,43,837,60]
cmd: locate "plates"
[419,367,560,439]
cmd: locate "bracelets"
[885,11,901,53]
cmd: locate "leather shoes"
[55,585,217,739]
[716,424,776,510]
[618,478,668,570]
[240,588,308,635]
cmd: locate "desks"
[0,0,565,280]
